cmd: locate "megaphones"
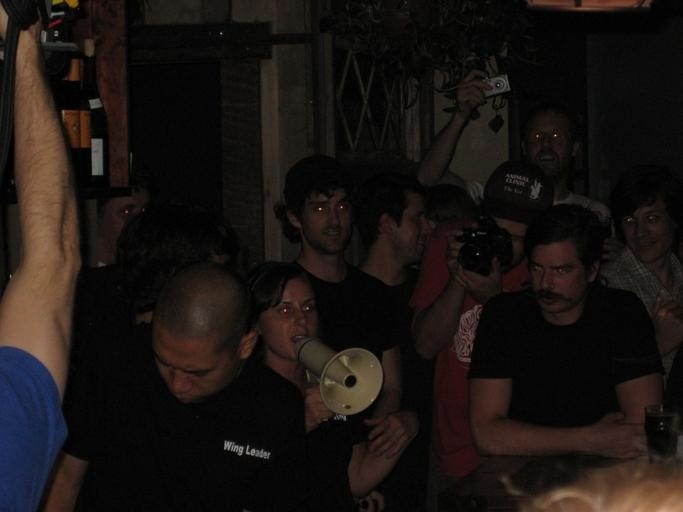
[293,337,383,416]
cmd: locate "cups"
[644,403,680,463]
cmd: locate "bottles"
[40,0,107,182]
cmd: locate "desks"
[432,453,626,511]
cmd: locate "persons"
[0,0,82,512]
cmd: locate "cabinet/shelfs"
[0,50,135,205]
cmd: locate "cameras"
[455,214,515,275]
[478,71,512,100]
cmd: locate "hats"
[480,158,557,228]
